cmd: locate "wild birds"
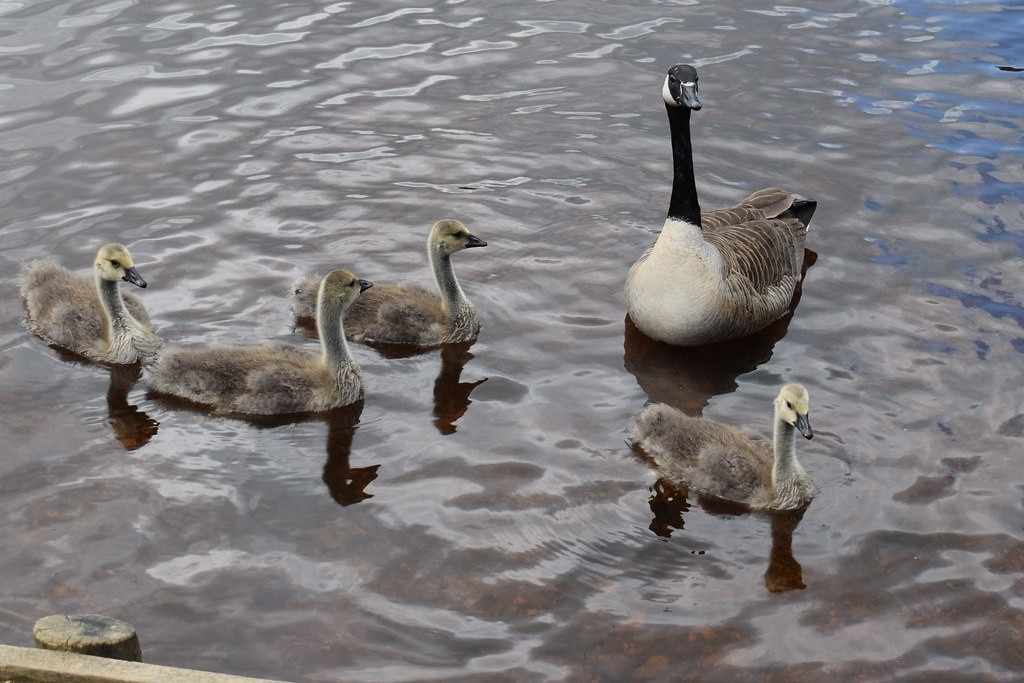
[15,217,492,419]
[623,62,820,349]
[622,383,819,512]
